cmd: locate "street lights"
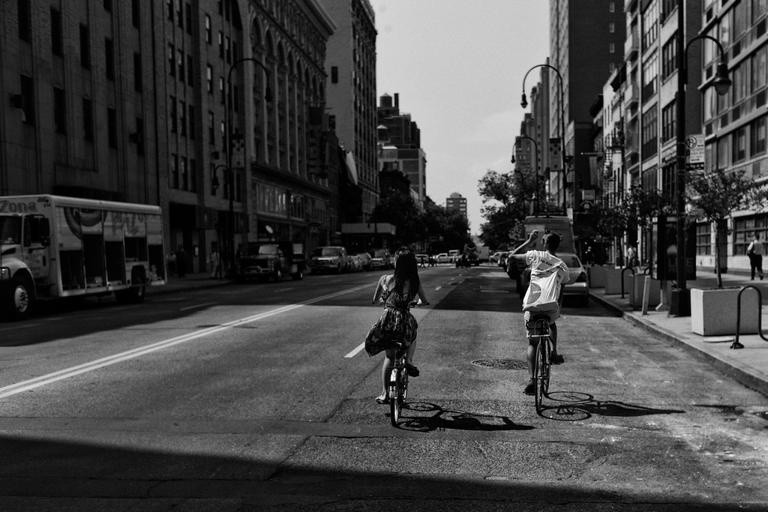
[510,136,540,218]
[520,63,569,213]
[212,56,275,283]
[668,1,731,318]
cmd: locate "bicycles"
[371,296,421,426]
[519,313,559,414]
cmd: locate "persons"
[210,249,222,277]
[747,233,765,281]
[166,249,177,278]
[626,243,638,266]
[371,245,431,404]
[419,255,438,269]
[507,228,573,397]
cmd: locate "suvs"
[311,245,347,272]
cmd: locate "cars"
[488,250,511,269]
[346,249,462,272]
[522,252,588,304]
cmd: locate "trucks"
[235,239,307,281]
[521,214,577,264]
[0,193,169,321]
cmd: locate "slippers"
[403,364,418,377]
[375,396,386,404]
